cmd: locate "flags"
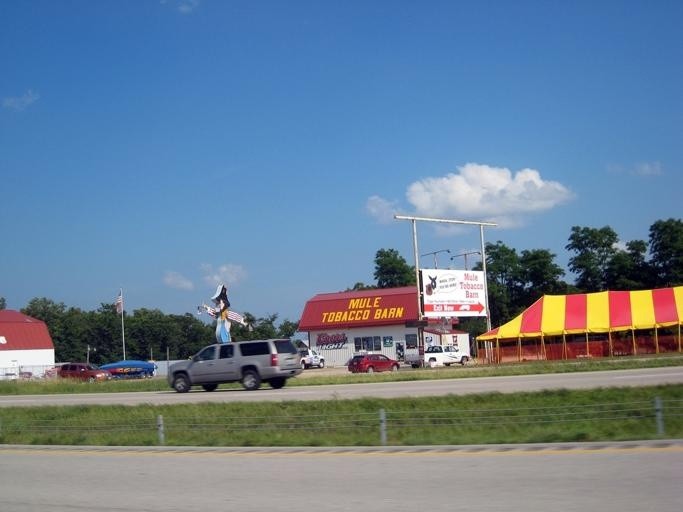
[115,290,122,314]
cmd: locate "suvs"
[61,364,112,383]
[296,348,325,369]
[168,337,304,393]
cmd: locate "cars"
[348,354,400,373]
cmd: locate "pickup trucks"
[404,346,471,367]
